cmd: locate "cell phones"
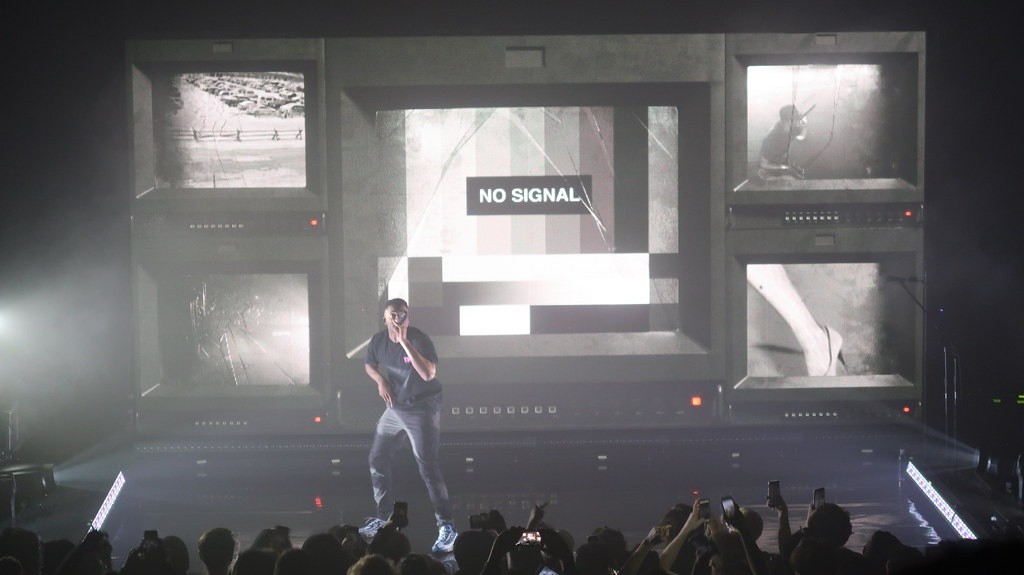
[813,487,825,510]
[768,480,780,507]
[721,496,735,518]
[144,529,159,556]
[699,499,710,520]
[393,501,407,527]
[275,526,288,538]
[469,515,491,529]
[520,531,541,545]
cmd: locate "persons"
[758,104,807,183]
[0,479,1024,575]
[357,298,459,555]
[746,263,852,376]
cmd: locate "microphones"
[887,276,920,283]
[394,312,407,328]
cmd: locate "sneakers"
[359,516,399,537]
[432,523,459,553]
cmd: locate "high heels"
[822,325,848,376]
